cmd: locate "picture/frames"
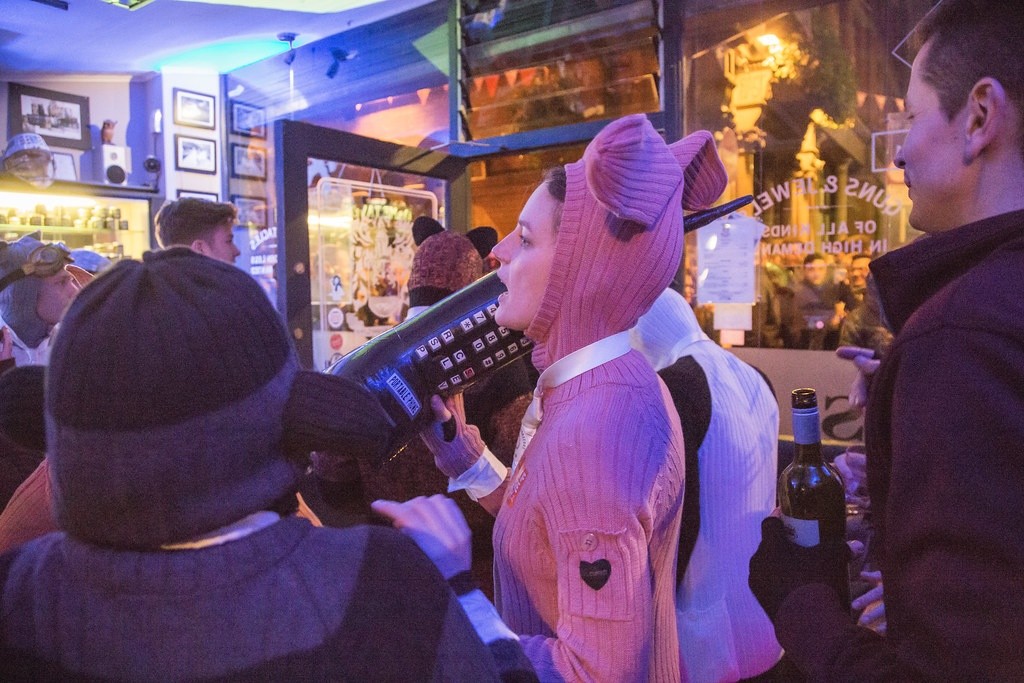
[176,188,219,203]
[230,142,268,182]
[231,192,268,231]
[174,134,217,175]
[52,151,78,182]
[230,99,267,140]
[7,81,93,151]
[172,87,216,131]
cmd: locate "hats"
[0,244,398,548]
[407,216,498,307]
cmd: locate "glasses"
[28,241,75,275]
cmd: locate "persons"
[301,217,535,600]
[373,114,727,683]
[155,197,241,265]
[0,246,540,683]
[633,288,786,683]
[746,0,1024,683]
[0,232,110,551]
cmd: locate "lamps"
[141,153,161,192]
[277,33,297,65]
[0,131,57,190]
[325,47,348,79]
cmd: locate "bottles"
[778,388,852,619]
